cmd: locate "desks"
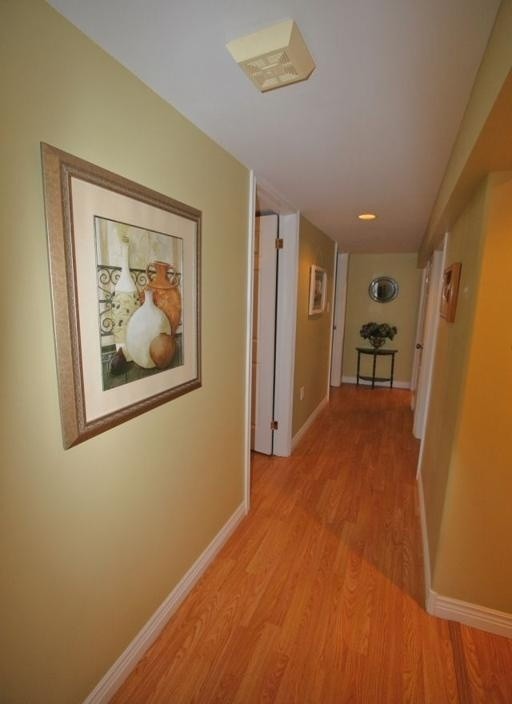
[356,347,398,389]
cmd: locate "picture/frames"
[367,276,399,303]
[41,141,202,450]
[308,265,327,316]
[438,262,462,322]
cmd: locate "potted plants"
[360,322,398,349]
